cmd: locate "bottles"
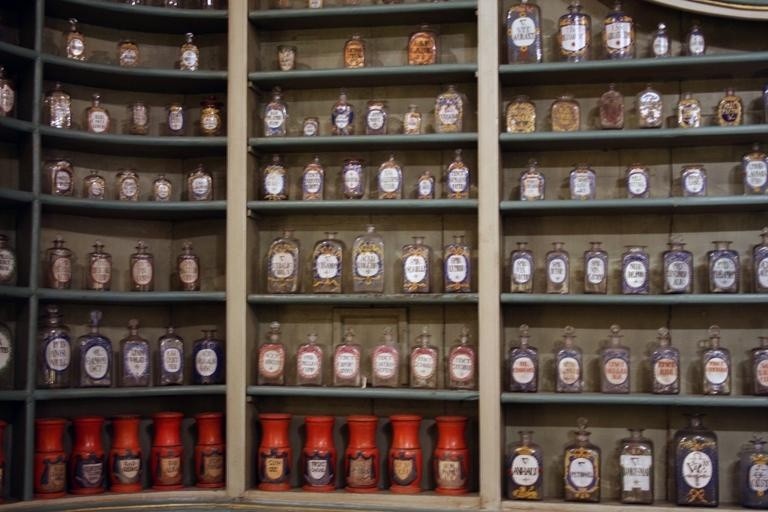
[255,413,470,494]
[43,80,226,202]
[116,38,143,68]
[264,148,471,198]
[0,417,14,499]
[0,66,18,117]
[177,33,200,72]
[47,235,201,292]
[509,416,767,511]
[0,323,14,387]
[0,233,19,288]
[344,32,368,69]
[62,18,88,63]
[265,221,472,292]
[125,0,221,10]
[34,304,225,497]
[513,145,766,203]
[516,323,767,396]
[503,84,744,133]
[259,319,477,391]
[263,83,472,132]
[274,45,298,73]
[507,229,767,294]
[407,22,442,65]
[508,1,706,64]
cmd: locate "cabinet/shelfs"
[487,0,767,510]
[239,2,487,509]
[1,1,239,507]
